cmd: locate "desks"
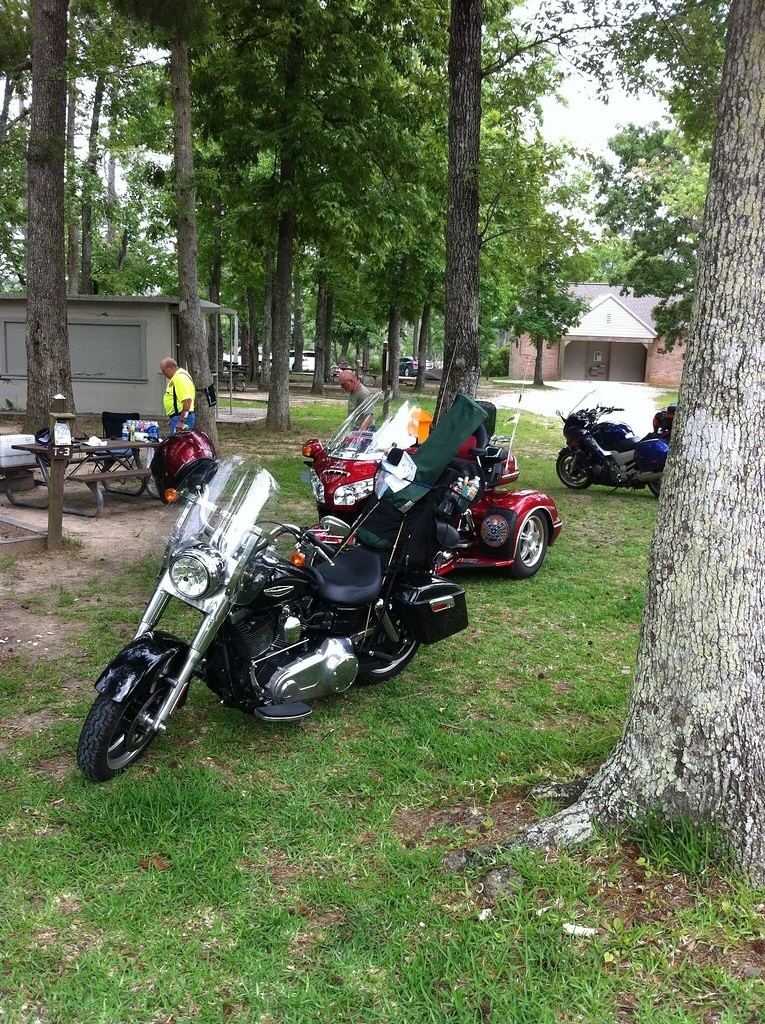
[209,370,243,391]
[11,439,161,518]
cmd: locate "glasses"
[341,380,349,386]
[162,366,170,372]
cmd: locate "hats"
[82,436,107,446]
[35,428,51,445]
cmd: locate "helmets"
[150,430,217,505]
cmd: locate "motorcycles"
[73,450,472,785]
[554,388,670,499]
[302,384,564,580]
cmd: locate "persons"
[160,357,196,435]
[338,370,377,437]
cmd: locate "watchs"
[180,419,185,423]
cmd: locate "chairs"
[91,411,143,482]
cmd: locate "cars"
[399,355,435,378]
[223,344,316,377]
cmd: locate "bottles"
[122,419,159,442]
[439,475,481,515]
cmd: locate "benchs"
[212,378,246,391]
[0,455,130,509]
[66,469,163,518]
[329,374,379,385]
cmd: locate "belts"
[170,412,191,419]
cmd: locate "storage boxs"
[395,576,467,647]
[0,434,36,467]
